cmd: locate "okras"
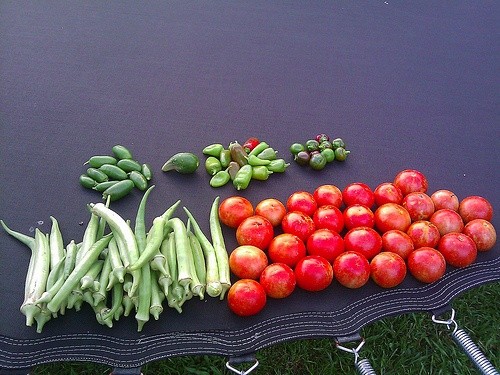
[0,185,232,333]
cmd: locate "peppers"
[79,133,350,202]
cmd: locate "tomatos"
[219,169,496,316]
